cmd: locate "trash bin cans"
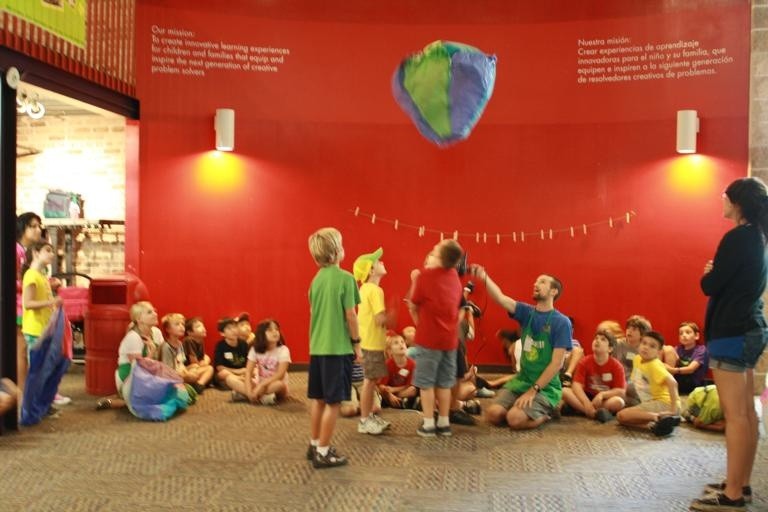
[83,274,150,395]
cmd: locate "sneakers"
[53,393,72,405]
[260,393,277,406]
[691,480,753,512]
[94,397,112,411]
[560,374,575,387]
[231,390,249,403]
[402,396,421,410]
[449,399,482,426]
[193,382,210,394]
[357,413,391,435]
[648,414,681,437]
[415,422,453,438]
[466,300,482,317]
[476,387,496,398]
[596,408,612,423]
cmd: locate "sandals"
[306,444,347,468]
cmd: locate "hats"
[353,246,384,282]
[233,312,250,323]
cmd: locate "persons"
[96,301,214,409]
[214,312,292,404]
[307,227,360,468]
[17,213,71,427]
[469,262,572,429]
[407,238,494,436]
[556,315,722,436]
[688,178,768,511]
[341,246,421,433]
[0,377,22,414]
[24,244,66,420]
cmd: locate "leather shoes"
[476,377,492,389]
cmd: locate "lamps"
[15,83,44,119]
[674,108,699,153]
[213,106,235,151]
